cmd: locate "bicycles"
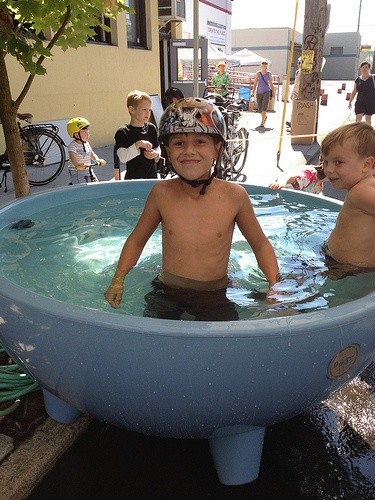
[157,86,249,178]
[0,113,65,192]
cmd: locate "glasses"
[262,63,268,64]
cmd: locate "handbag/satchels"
[269,89,273,99]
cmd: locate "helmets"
[158,97,227,144]
[67,117,91,138]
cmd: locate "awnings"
[231,47,271,65]
[179,39,228,61]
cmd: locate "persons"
[67,116,105,184]
[266,162,323,191]
[285,56,303,127]
[320,122,375,272]
[250,61,275,127]
[211,61,231,97]
[104,97,280,321]
[348,61,375,125]
[114,89,161,180]
